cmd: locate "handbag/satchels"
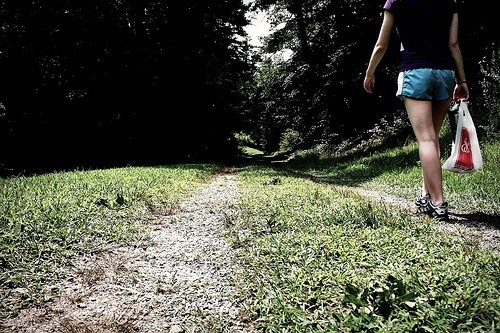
[442,99,483,174]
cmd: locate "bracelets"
[455,80,467,85]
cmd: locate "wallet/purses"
[446,102,459,143]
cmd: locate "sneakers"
[416,200,449,221]
[413,193,430,207]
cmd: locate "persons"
[362,1,470,220]
